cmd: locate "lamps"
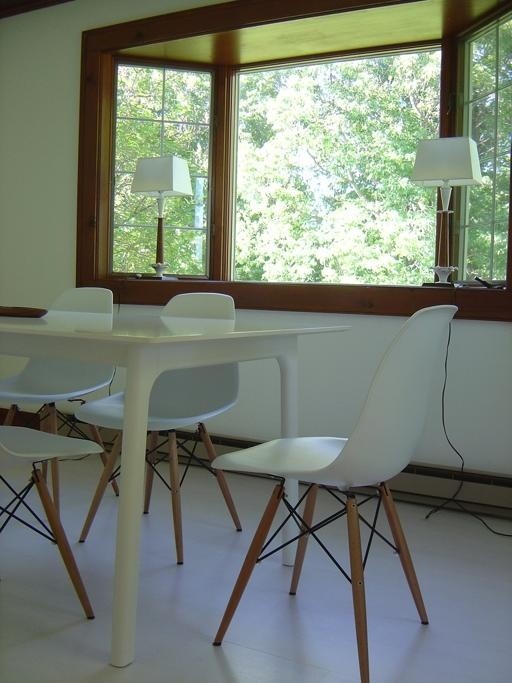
[131,153,194,280]
[407,135,483,287]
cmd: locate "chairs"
[0,283,118,513]
[73,290,242,567]
[1,424,106,619]
[207,302,460,683]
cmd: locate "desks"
[1,309,354,669]
